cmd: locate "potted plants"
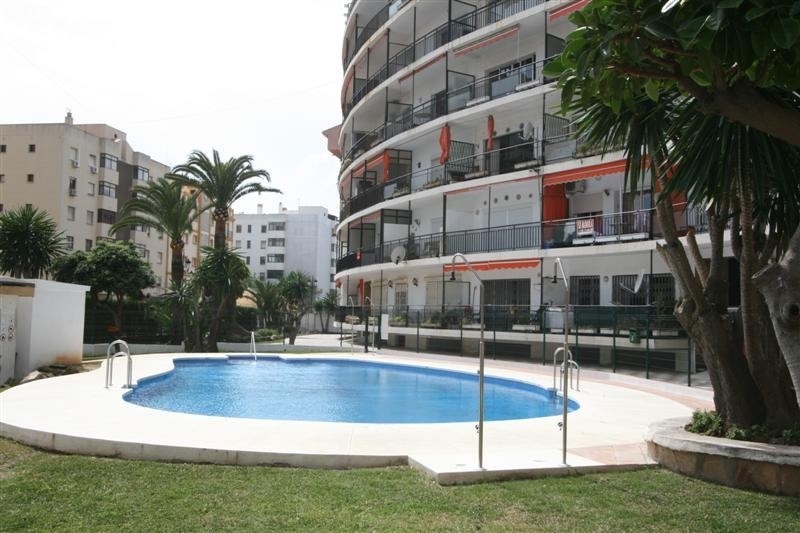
[462,317,487,330]
[425,177,445,188]
[576,139,630,159]
[421,312,442,328]
[393,183,410,197]
[445,310,463,328]
[390,312,413,326]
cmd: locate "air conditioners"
[565,181,585,195]
[91,166,98,174]
[73,161,80,168]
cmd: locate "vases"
[465,169,487,179]
[573,233,650,247]
[466,95,490,109]
[515,159,541,170]
[513,324,541,334]
[516,80,542,93]
